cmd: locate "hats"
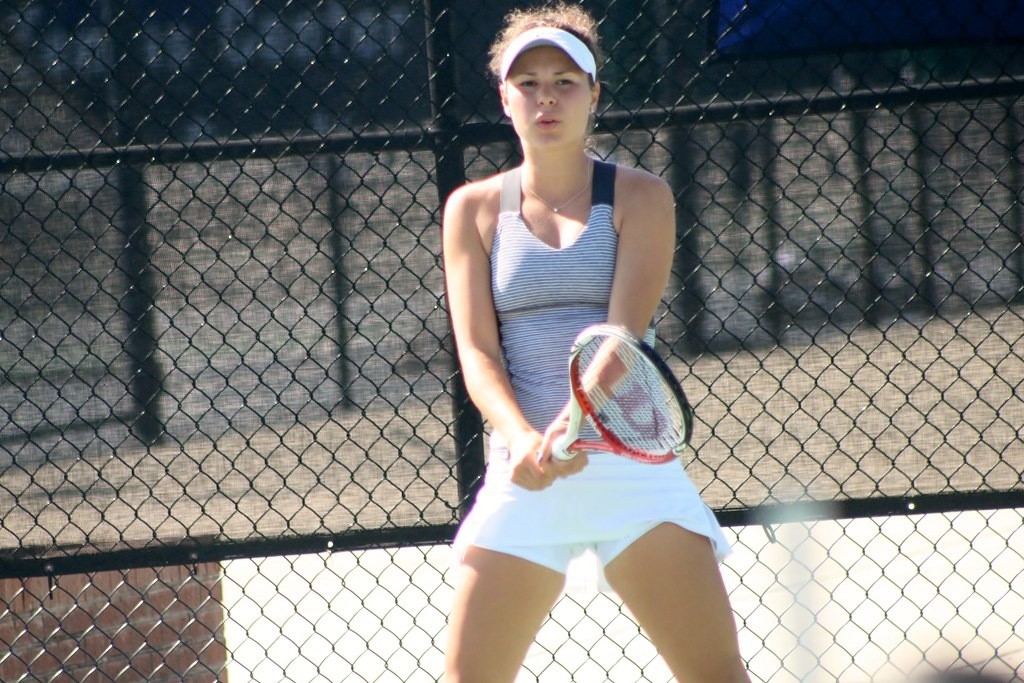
[500,27,597,84]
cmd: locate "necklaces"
[522,157,591,213]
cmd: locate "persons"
[441,0,754,683]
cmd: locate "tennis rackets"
[548,322,693,463]
